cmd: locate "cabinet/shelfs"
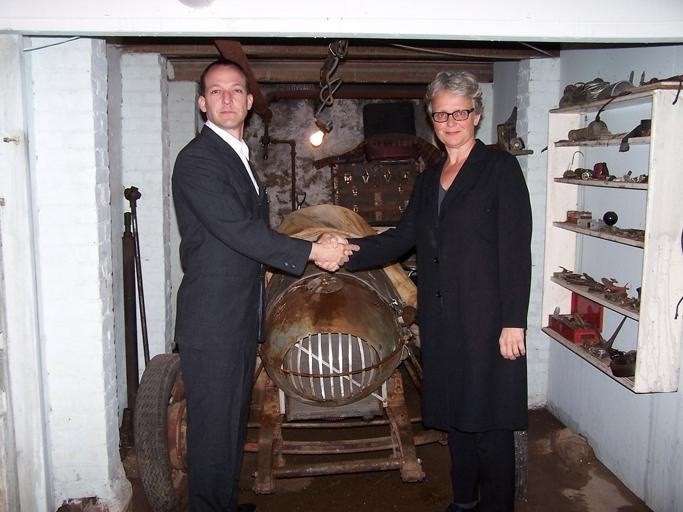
[539,83,682,395]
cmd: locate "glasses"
[432,104,474,123]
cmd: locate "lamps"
[308,56,341,148]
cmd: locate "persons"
[171,59,361,512]
[312,71,532,512]
